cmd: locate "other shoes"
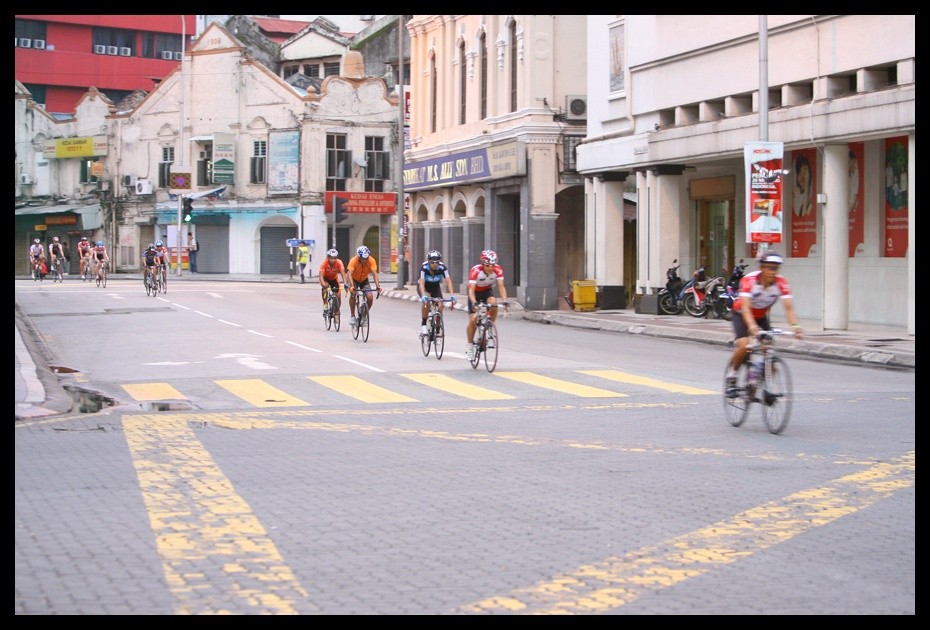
[421,325,428,335]
[334,312,339,322]
[350,316,356,324]
[323,303,328,311]
[726,371,737,397]
[466,343,475,361]
[30,265,107,280]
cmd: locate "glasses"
[361,258,369,260]
[430,260,439,264]
[482,264,494,267]
[762,265,777,270]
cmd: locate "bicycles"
[321,283,348,331]
[80,255,93,282]
[723,327,792,435]
[419,295,457,359]
[348,286,381,344]
[143,263,167,297]
[50,257,65,283]
[32,256,47,282]
[466,299,509,373]
[94,260,110,287]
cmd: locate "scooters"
[658,258,748,319]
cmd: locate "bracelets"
[791,323,800,331]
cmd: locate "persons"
[790,147,908,216]
[30,225,208,299]
[725,251,804,382]
[416,250,458,346]
[466,249,511,362]
[296,241,312,283]
[402,234,411,285]
[318,249,348,324]
[347,245,384,325]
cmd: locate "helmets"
[327,248,338,256]
[155,240,163,247]
[97,241,104,246]
[428,250,442,259]
[148,243,154,250]
[357,246,370,258]
[81,237,87,241]
[34,238,40,243]
[480,250,498,266]
[53,236,59,242]
[759,252,784,263]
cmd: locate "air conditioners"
[135,180,154,196]
[563,137,587,171]
[563,93,588,122]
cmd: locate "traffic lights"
[336,197,349,224]
[182,197,195,223]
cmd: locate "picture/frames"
[606,17,631,101]
